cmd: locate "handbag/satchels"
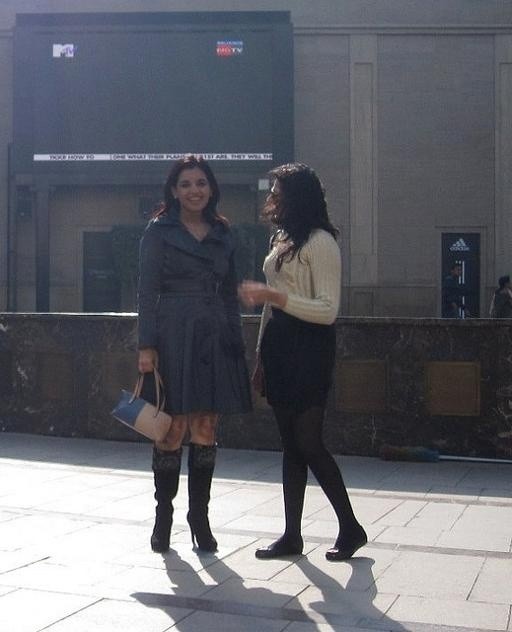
[112,370,172,442]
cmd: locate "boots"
[188,442,218,551]
[152,448,183,550]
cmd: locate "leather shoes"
[326,527,366,559]
[256,539,303,559]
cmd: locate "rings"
[248,297,256,304]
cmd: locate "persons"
[135,152,239,555]
[239,161,370,562]
[442,262,465,318]
[489,275,511,318]
